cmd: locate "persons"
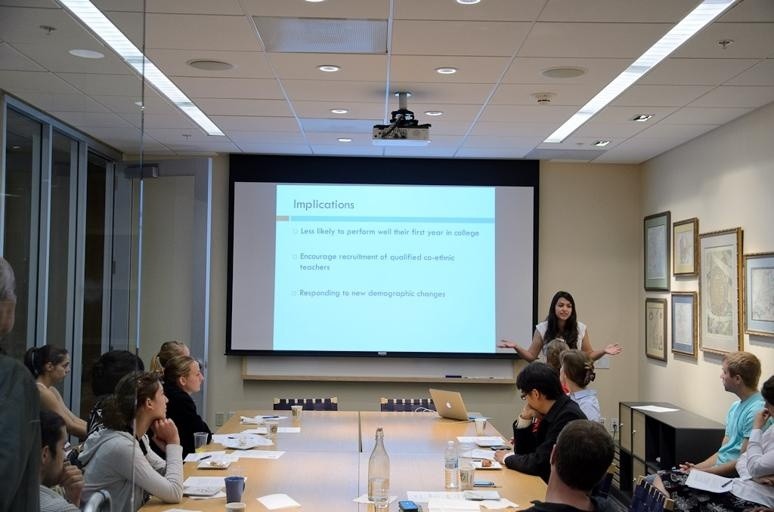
[649,351,773,512]
[0,261,212,512]
[494,290,623,512]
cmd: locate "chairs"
[627,476,674,512]
[380,397,437,411]
[272,397,338,411]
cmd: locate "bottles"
[444,441,459,489]
[369,426,391,503]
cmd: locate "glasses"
[521,394,527,400]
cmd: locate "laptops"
[429,388,487,422]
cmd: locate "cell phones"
[491,445,511,450]
[473,480,496,487]
[399,501,418,512]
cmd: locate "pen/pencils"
[722,480,732,487]
[200,455,211,460]
[263,416,278,418]
[228,437,252,439]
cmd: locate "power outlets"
[608,418,618,432]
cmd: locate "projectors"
[371,92,432,147]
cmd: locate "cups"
[475,419,487,435]
[193,432,208,454]
[267,422,278,437]
[292,406,302,418]
[460,466,474,490]
[224,476,246,512]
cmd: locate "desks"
[137,410,551,512]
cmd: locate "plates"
[476,440,505,447]
[473,461,504,470]
[465,490,500,501]
[198,462,232,469]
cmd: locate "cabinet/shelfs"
[618,401,725,497]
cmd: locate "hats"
[761,375,774,406]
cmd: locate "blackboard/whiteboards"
[241,355,517,386]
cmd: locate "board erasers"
[446,375,462,378]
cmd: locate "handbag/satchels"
[92,350,144,395]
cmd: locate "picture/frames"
[672,217,699,277]
[644,211,671,291]
[743,251,774,337]
[670,290,697,357]
[644,297,667,363]
[697,226,744,357]
